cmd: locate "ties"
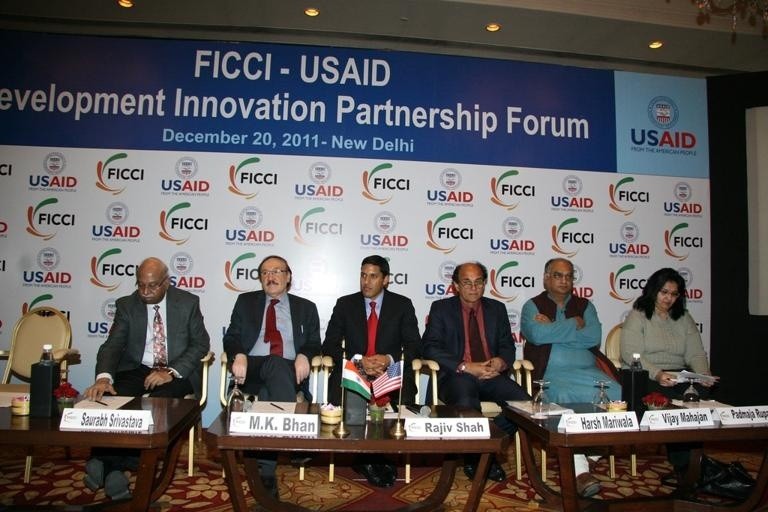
[364,302,378,357]
[263,299,282,358]
[467,308,486,363]
[152,305,167,371]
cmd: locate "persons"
[521,258,623,498]
[321,255,422,488]
[620,267,726,488]
[223,255,321,501]
[82,257,210,501]
[421,262,533,482]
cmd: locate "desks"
[0,398,202,511]
[207,405,509,512]
[502,400,768,511]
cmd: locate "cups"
[369,404,386,424]
[57,397,74,413]
[228,376,245,415]
[11,401,30,416]
[320,409,343,425]
[370,407,385,426]
[609,403,627,413]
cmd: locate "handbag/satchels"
[700,454,755,504]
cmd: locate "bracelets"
[164,368,176,380]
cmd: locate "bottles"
[40,344,55,367]
[631,353,643,371]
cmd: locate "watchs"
[354,354,362,366]
[458,361,465,375]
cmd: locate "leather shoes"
[485,462,505,483]
[379,461,398,485]
[661,473,680,487]
[575,473,600,498]
[462,457,476,481]
[104,469,133,503]
[264,475,279,502]
[586,456,596,472]
[82,452,104,492]
[362,463,390,489]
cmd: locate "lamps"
[666,0,767,45]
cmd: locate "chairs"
[220,350,321,481]
[0,306,72,483]
[420,358,522,482]
[519,352,615,482]
[322,356,422,484]
[142,351,214,477]
[606,324,638,478]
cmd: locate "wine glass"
[592,379,610,411]
[683,377,700,408]
[534,380,551,420]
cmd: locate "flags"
[340,360,371,401]
[371,360,404,399]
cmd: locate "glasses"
[658,288,680,298]
[548,272,573,281]
[259,268,286,276]
[456,277,484,289]
[134,275,168,290]
